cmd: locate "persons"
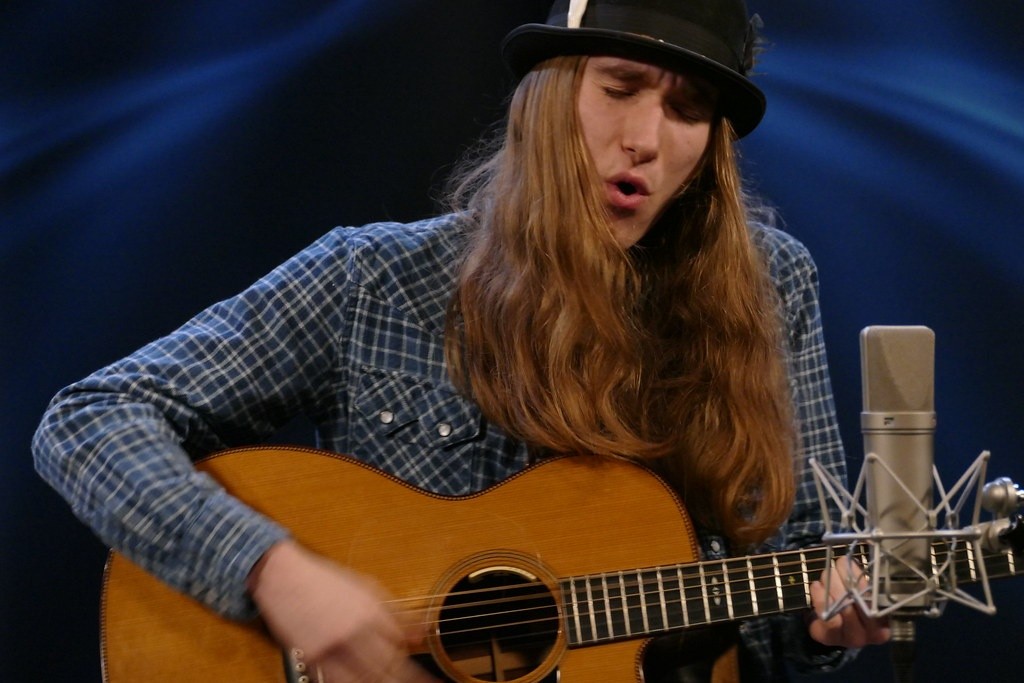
[31,0,892,683]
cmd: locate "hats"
[498,0,766,141]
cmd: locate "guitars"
[97,442,1023,683]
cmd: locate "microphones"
[859,323,937,671]
